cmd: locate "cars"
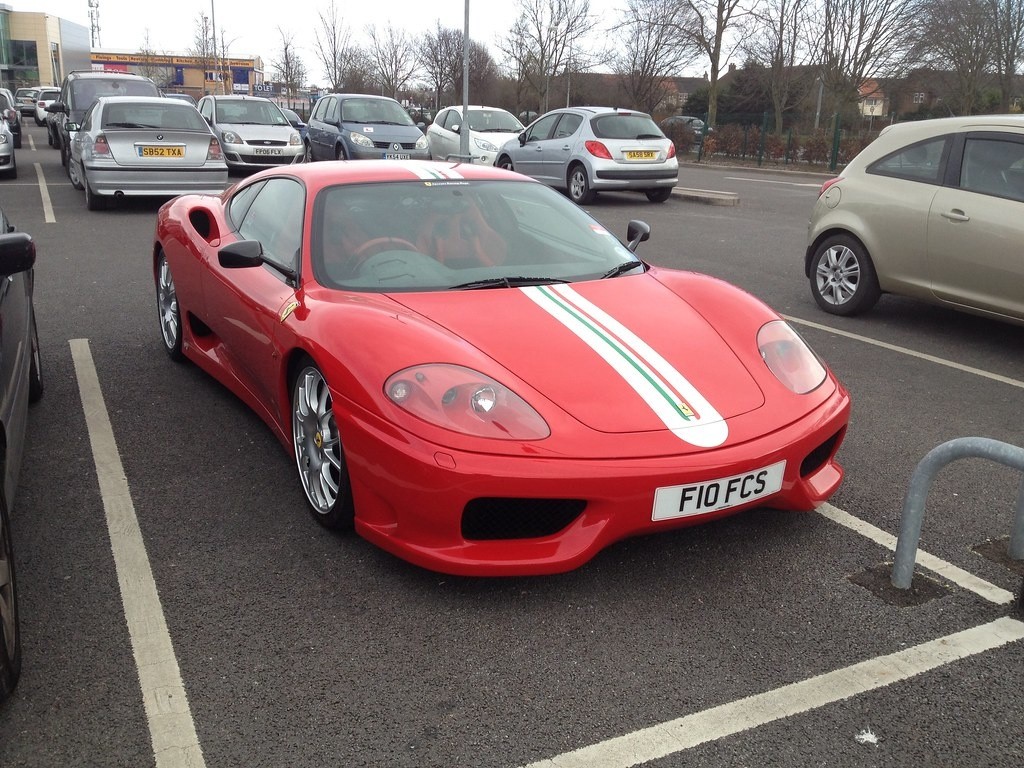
[279,109,307,134]
[426,103,528,168]
[494,105,679,205]
[0,205,45,699]
[804,111,1023,328]
[196,94,305,171]
[407,108,431,126]
[301,93,432,164]
[518,111,539,128]
[64,94,229,209]
[656,116,714,143]
[162,93,198,109]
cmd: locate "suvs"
[0,68,168,179]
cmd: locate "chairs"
[217,108,225,122]
[276,201,377,280]
[903,146,928,174]
[126,85,148,95]
[965,141,1007,191]
[76,83,97,109]
[160,111,189,129]
[416,197,509,269]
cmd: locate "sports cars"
[149,157,853,580]
